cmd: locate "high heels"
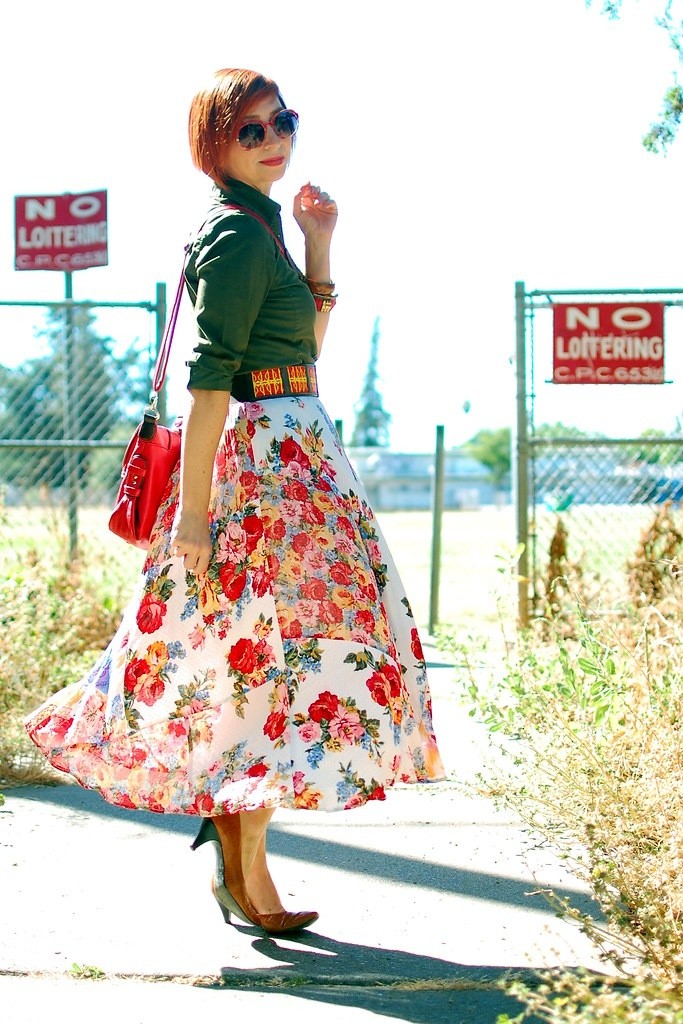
[191,812,262,926]
[212,873,320,934]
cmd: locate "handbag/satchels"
[109,415,181,549]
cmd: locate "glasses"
[233,108,300,152]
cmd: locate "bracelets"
[305,277,337,313]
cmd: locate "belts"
[230,365,320,402]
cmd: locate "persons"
[24,69,448,933]
